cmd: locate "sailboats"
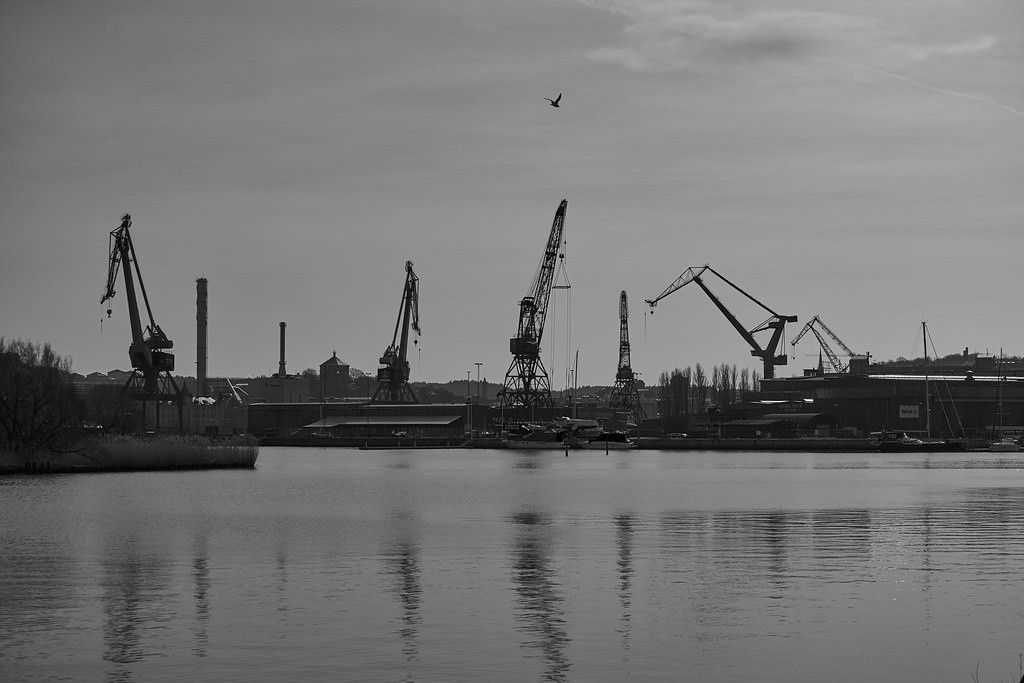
[869,320,974,454]
[986,348,1024,453]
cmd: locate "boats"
[457,392,639,451]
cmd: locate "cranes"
[601,289,640,411]
[100,212,188,435]
[498,197,573,423]
[363,259,422,406]
[790,312,859,373]
[643,264,798,379]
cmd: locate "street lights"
[569,370,574,402]
[474,363,483,404]
[466,371,471,400]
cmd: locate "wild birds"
[544,93,562,108]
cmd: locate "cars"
[393,431,408,438]
